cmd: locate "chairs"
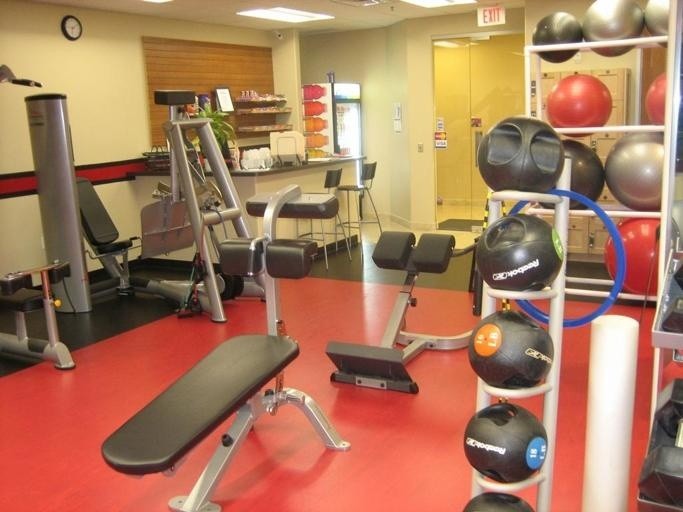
[297,161,384,272]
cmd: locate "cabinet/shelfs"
[528,69,629,256]
[233,93,293,133]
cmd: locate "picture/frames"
[215,86,233,113]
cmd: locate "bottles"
[303,85,328,159]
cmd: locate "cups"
[327,71,336,82]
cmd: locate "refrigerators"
[304,83,363,185]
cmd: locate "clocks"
[61,15,82,41]
